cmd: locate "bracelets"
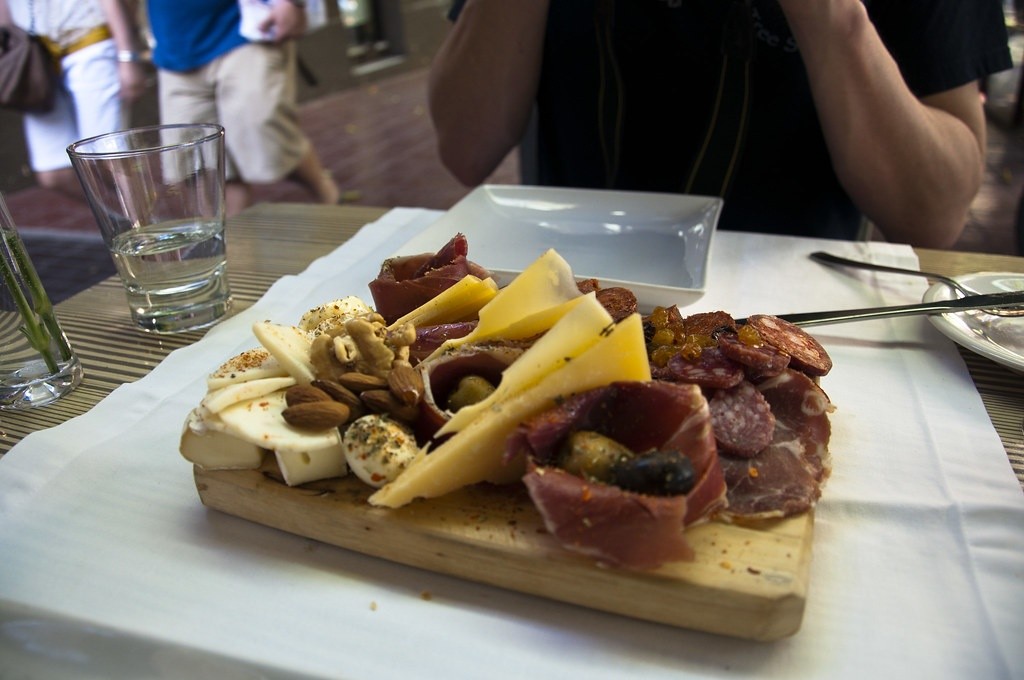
[117,51,139,63]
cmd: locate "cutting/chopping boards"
[195,313,823,643]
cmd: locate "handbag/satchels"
[238,0,328,41]
[0,24,57,115]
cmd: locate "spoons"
[809,250,1024,320]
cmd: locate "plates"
[403,179,724,313]
[919,269,1024,377]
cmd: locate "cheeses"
[388,246,597,363]
[175,293,375,487]
[403,295,618,469]
[367,313,650,507]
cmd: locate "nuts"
[280,313,426,428]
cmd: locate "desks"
[1,205,1022,680]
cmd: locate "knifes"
[724,288,1024,331]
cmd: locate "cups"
[0,191,84,408]
[64,122,234,334]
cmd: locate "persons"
[142,0,340,215]
[425,0,1013,251]
[0,1,140,211]
